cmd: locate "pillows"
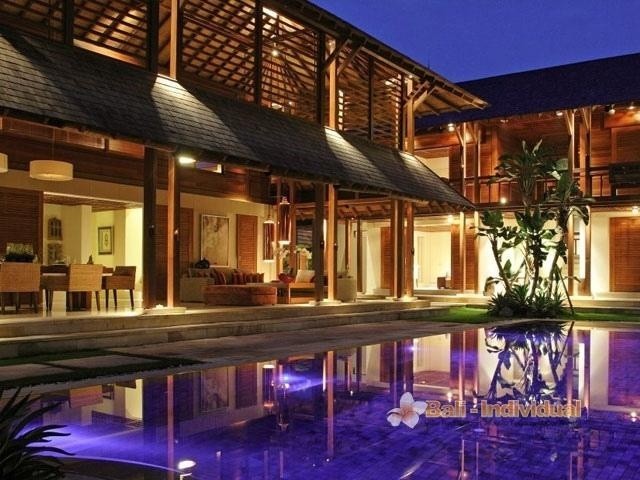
[196,269,248,285]
[278,269,328,286]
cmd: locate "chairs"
[66,379,136,410]
[0,261,137,313]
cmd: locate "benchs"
[200,285,278,306]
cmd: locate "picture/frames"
[97,226,112,255]
[199,214,230,268]
[198,365,229,414]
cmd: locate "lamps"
[264,205,274,224]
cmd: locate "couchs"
[271,272,354,299]
[179,266,264,305]
